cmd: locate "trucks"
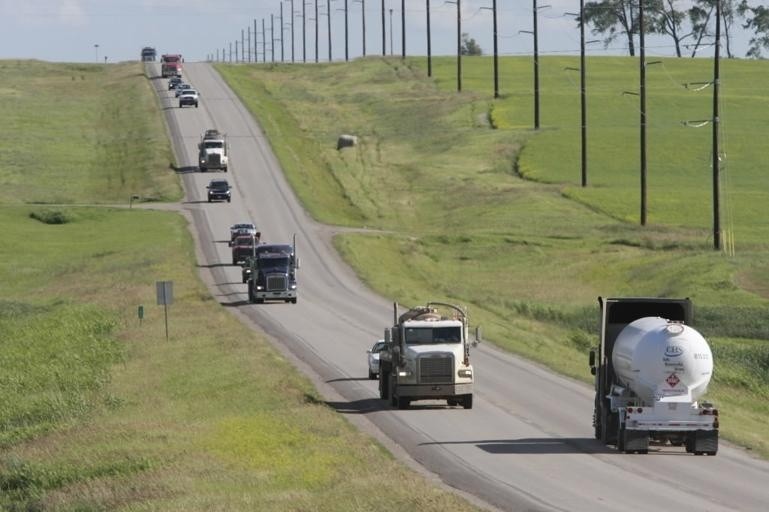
[588,295,720,457]
[161,54,184,78]
[378,300,474,410]
[242,235,299,304]
[198,130,228,174]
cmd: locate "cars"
[228,223,262,265]
[207,179,232,204]
[366,339,393,379]
[168,79,199,108]
